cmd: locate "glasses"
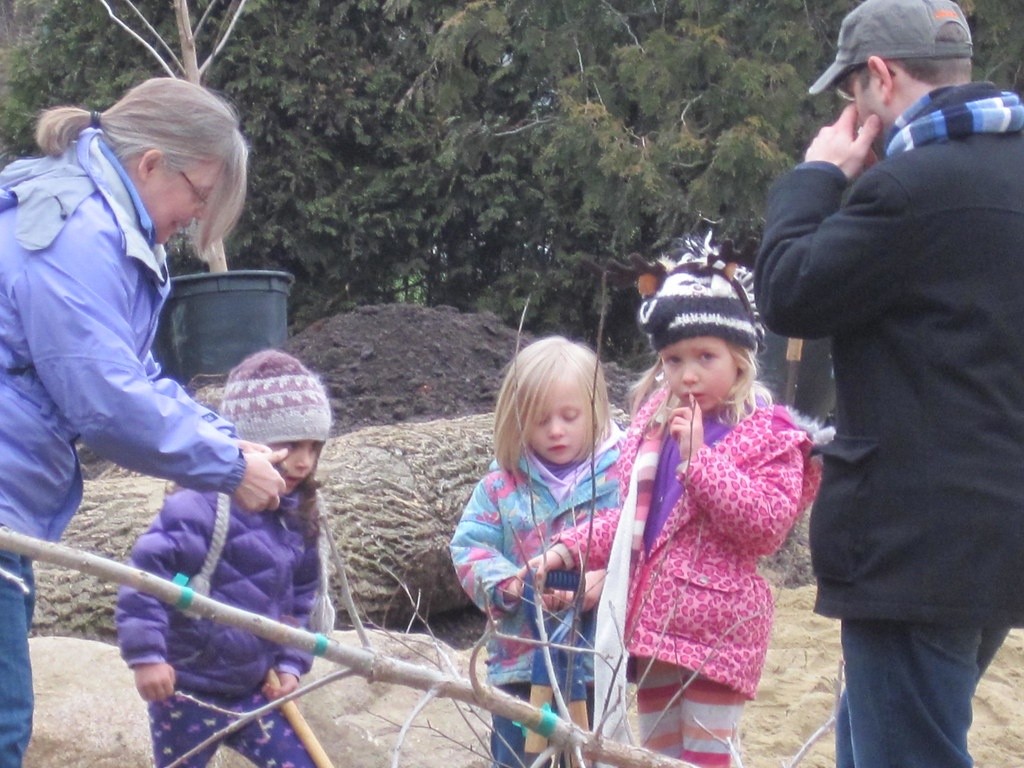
[179,168,212,205]
[833,54,896,100]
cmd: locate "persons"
[0,77,288,768]
[753,0,1024,768]
[114,347,335,768]
[516,247,833,768]
[450,337,628,768]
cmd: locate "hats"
[637,231,766,356]
[174,352,335,633]
[810,0,974,96]
